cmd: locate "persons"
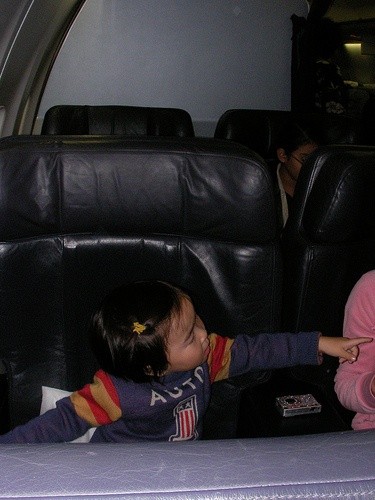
[267,126,327,231]
[334,269,375,431]
[0,279,374,445]
[310,40,353,145]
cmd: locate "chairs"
[0,106,375,445]
